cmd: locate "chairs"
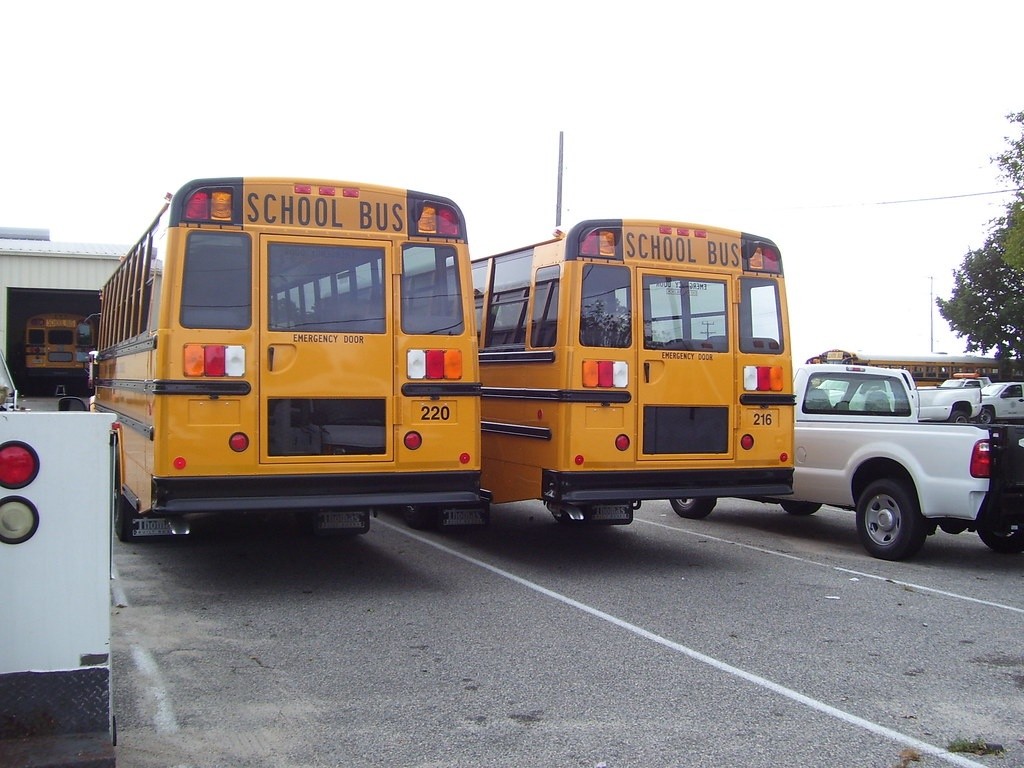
[863,390,892,412]
[806,388,832,409]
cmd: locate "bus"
[26,313,98,396]
[324,219,796,532]
[75,175,493,553]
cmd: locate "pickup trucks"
[666,361,1024,562]
[817,375,1023,426]
